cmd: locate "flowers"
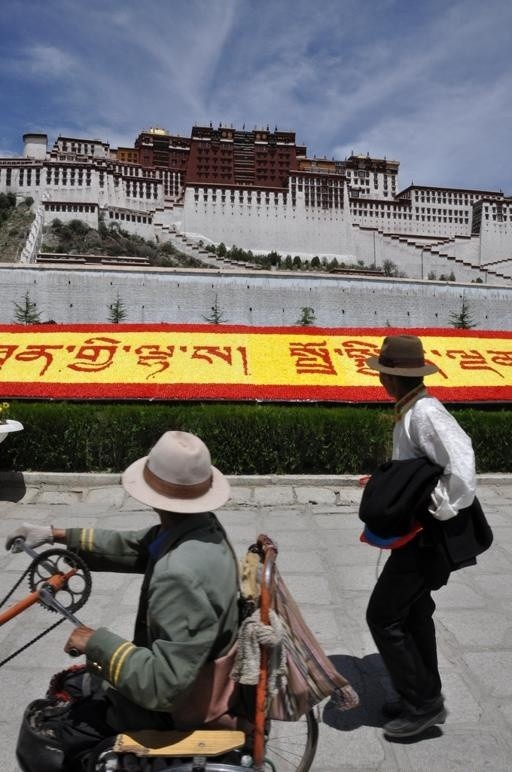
[0,402,10,424]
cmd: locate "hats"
[366,335,439,377]
[121,429,232,516]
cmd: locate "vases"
[0,418,25,442]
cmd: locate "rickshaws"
[3,533,319,770]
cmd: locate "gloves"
[3,523,54,552]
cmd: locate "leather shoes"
[382,697,447,738]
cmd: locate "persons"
[358,332,477,739]
[3,429,240,771]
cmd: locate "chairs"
[71,534,279,770]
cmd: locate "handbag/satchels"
[263,563,351,722]
